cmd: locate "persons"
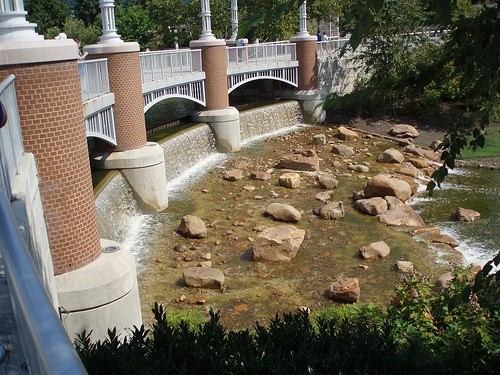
[316,31,322,44]
[322,32,329,48]
